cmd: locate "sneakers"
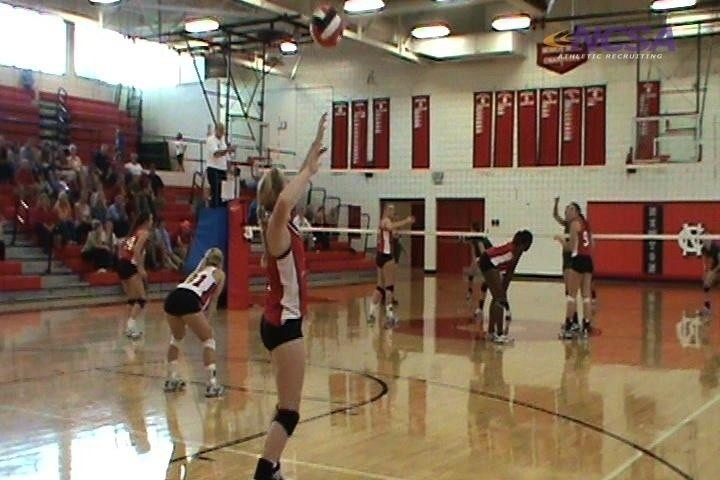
[367,298,398,329]
[475,306,515,342]
[205,385,224,398]
[124,326,143,338]
[565,323,593,338]
[163,378,186,391]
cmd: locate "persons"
[696,232,720,315]
[247,194,329,241]
[164,248,227,397]
[206,123,236,208]
[480,230,534,344]
[118,211,154,339]
[254,112,328,480]
[466,220,512,321]
[367,202,415,328]
[553,195,596,330]
[0,133,191,274]
[553,201,601,340]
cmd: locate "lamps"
[279,38,297,56]
[491,13,531,32]
[184,16,220,32]
[410,21,452,39]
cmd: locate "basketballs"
[311,3,342,46]
